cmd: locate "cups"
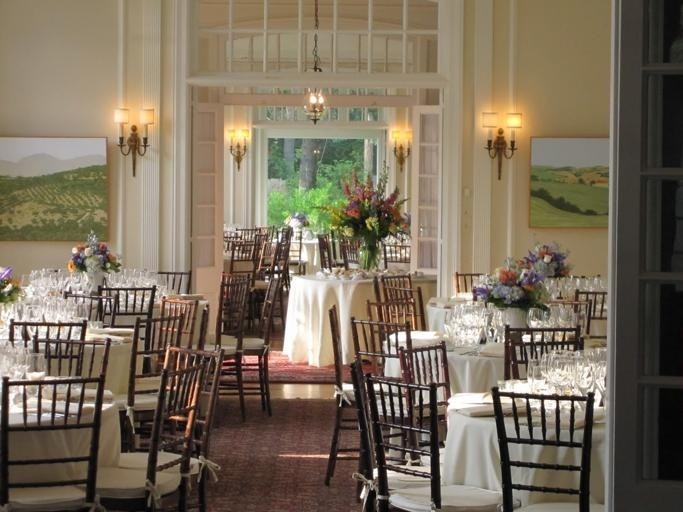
[1,299,104,340]
[26,266,168,303]
[0,340,53,393]
[315,267,422,281]
[544,273,608,308]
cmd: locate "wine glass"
[521,344,608,416]
[443,300,577,354]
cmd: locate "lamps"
[226,126,251,173]
[389,127,413,172]
[480,108,522,185]
[301,0,331,126]
[109,106,155,179]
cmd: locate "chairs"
[324,271,609,512]
[220,227,436,368]
[1,269,280,512]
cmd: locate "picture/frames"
[0,131,111,247]
[525,134,609,232]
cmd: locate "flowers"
[517,241,569,278]
[299,156,415,274]
[473,260,550,310]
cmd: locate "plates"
[107,329,134,336]
[180,294,202,300]
